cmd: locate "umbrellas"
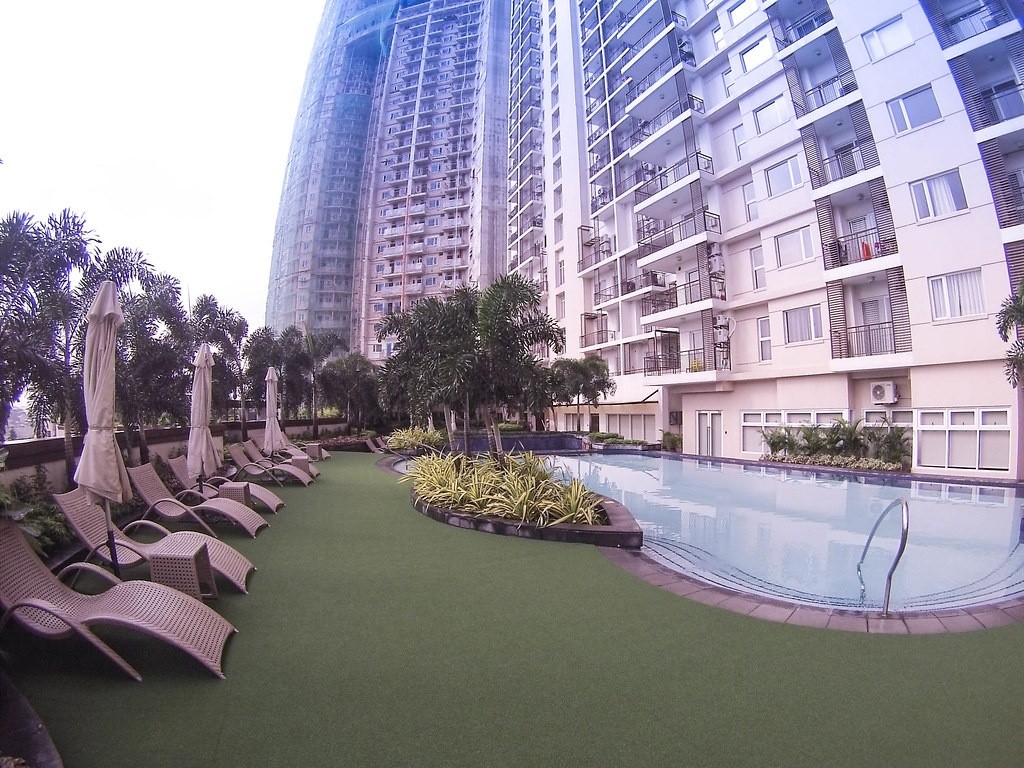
[264,366,287,485]
[74,281,133,582]
[187,342,222,523]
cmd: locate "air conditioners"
[600,243,610,253]
[680,44,693,58]
[600,234,609,245]
[713,315,725,327]
[643,163,654,174]
[708,255,725,274]
[597,187,605,197]
[677,34,688,47]
[651,297,660,307]
[870,381,897,404]
[644,219,657,233]
[713,329,728,343]
[638,119,649,127]
[707,243,721,256]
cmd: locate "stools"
[218,482,253,510]
[306,443,322,459]
[149,539,220,603]
[291,455,309,481]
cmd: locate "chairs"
[52,487,257,594]
[365,438,384,453]
[0,518,239,683]
[242,439,322,478]
[281,431,332,460]
[375,437,387,448]
[227,443,315,488]
[282,444,314,463]
[125,462,270,539]
[167,455,287,514]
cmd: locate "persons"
[541,418,550,431]
